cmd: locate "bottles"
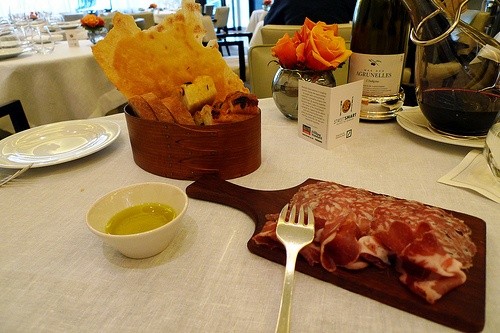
[344,0,412,122]
[410,0,500,139]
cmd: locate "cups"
[0,9,66,59]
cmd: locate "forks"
[274,203,314,333]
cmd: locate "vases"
[87,28,106,45]
[271,68,336,121]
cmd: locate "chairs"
[203,4,353,100]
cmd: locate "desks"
[0,21,130,132]
[0,97,500,333]
[152,13,178,25]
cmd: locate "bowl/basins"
[85,182,189,260]
[123,103,262,181]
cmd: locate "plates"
[396,107,486,148]
[0,119,121,168]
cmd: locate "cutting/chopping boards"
[184,177,486,333]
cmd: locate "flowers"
[269,19,352,69]
[82,15,107,32]
